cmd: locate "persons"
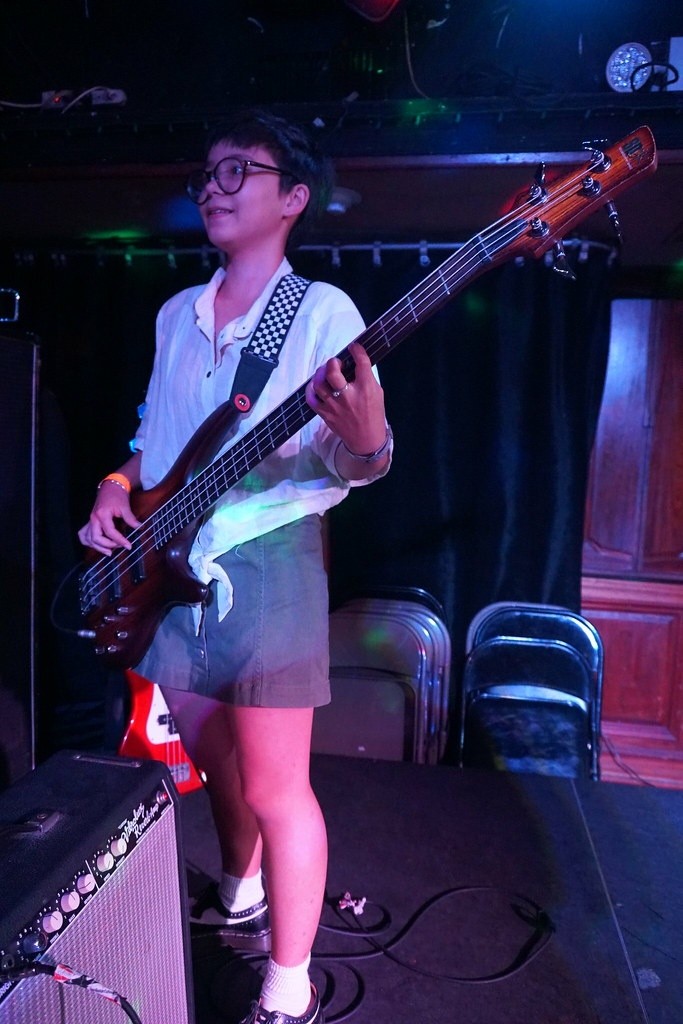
[76,114,399,1024]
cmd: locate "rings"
[332,383,350,398]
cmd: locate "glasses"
[187,156,296,204]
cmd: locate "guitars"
[77,124,663,672]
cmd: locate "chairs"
[312,584,454,763]
[456,601,605,781]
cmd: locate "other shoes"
[189,881,271,939]
[239,981,325,1024]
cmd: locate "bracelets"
[98,473,131,494]
[342,425,393,463]
